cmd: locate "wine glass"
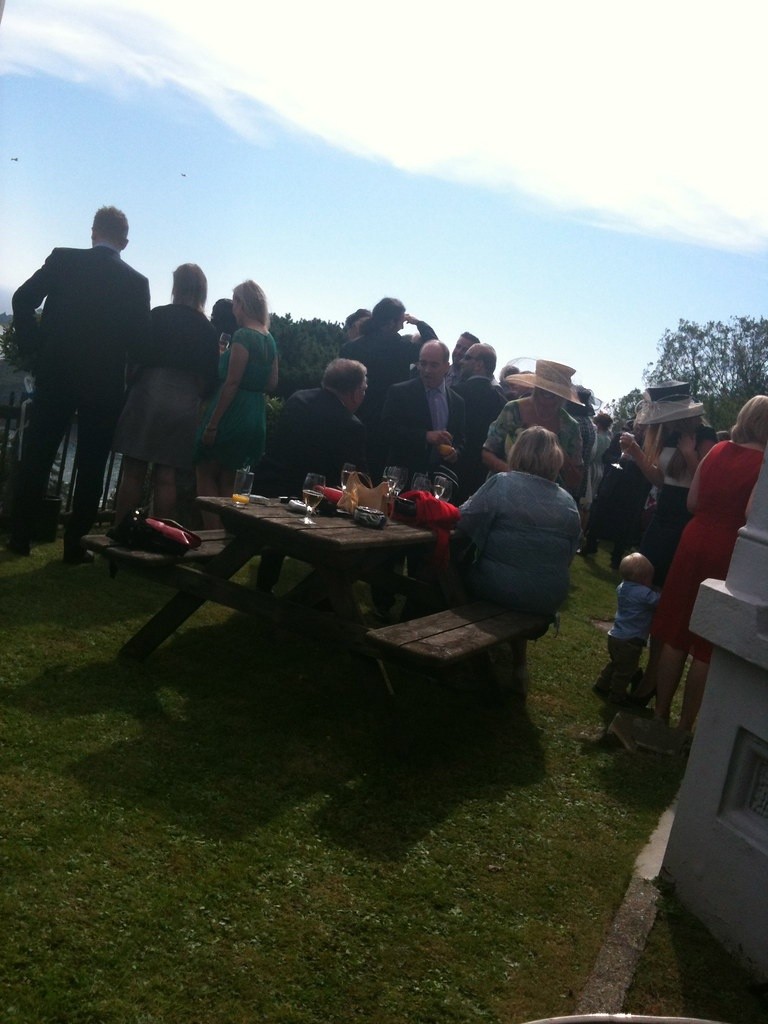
[611,434,635,470]
[299,473,326,524]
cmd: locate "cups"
[383,467,453,502]
[220,333,232,352]
[232,470,254,508]
[440,440,450,456]
[341,463,356,492]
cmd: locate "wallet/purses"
[309,483,343,516]
[394,495,416,516]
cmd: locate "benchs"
[81,530,262,568]
[367,603,555,664]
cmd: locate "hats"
[564,385,602,416]
[506,360,586,407]
[635,381,706,425]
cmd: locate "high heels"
[623,680,656,709]
[629,667,643,693]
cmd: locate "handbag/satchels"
[337,472,389,517]
[106,507,201,557]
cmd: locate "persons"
[10,207,768,764]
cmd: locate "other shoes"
[608,694,631,708]
[610,554,622,569]
[63,549,94,564]
[592,683,610,697]
[370,595,392,619]
[5,538,31,557]
[576,541,599,554]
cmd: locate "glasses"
[463,354,478,361]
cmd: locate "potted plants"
[0,313,62,542]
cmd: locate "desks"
[120,496,501,754]
